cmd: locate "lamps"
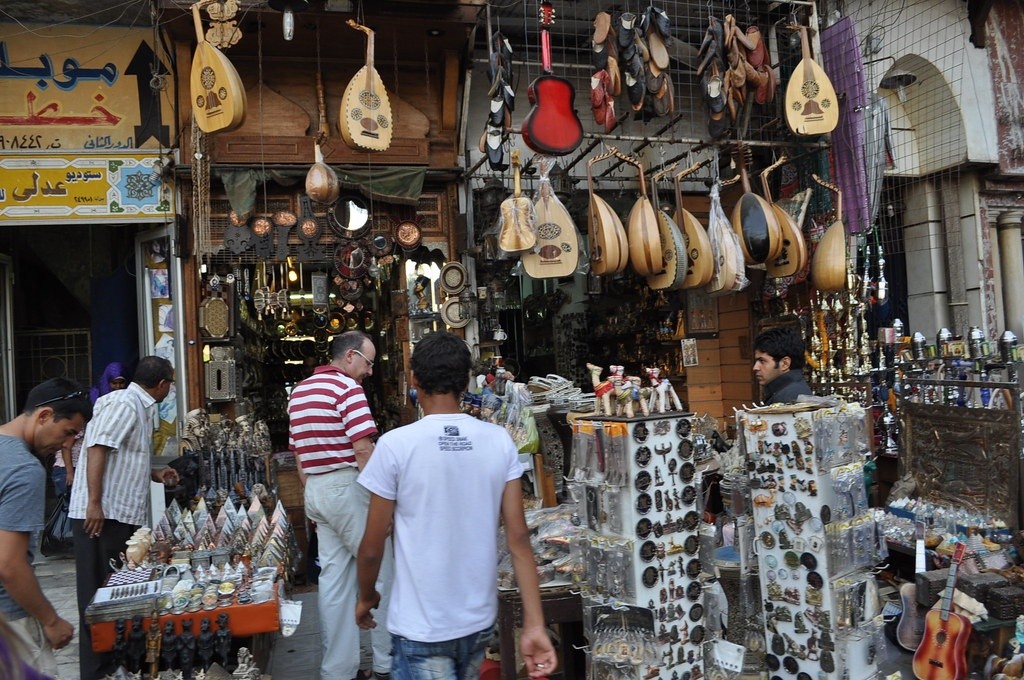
[862,56,916,102]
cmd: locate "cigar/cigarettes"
[95,533,99,536]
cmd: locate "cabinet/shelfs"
[376,221,1024,680]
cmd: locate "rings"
[537,664,544,668]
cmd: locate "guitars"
[895,521,932,653]
[911,541,973,680]
[520,0,585,158]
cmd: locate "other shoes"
[478,31,517,170]
[586,7,775,139]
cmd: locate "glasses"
[34,389,90,406]
[164,378,176,385]
[353,350,374,368]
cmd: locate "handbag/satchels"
[40,484,74,557]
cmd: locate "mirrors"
[341,246,365,269]
[373,235,388,249]
[333,196,369,231]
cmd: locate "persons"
[753,327,813,407]
[65,356,180,680]
[87,361,127,407]
[287,331,395,680]
[50,422,87,501]
[354,332,558,680]
[0,379,94,680]
